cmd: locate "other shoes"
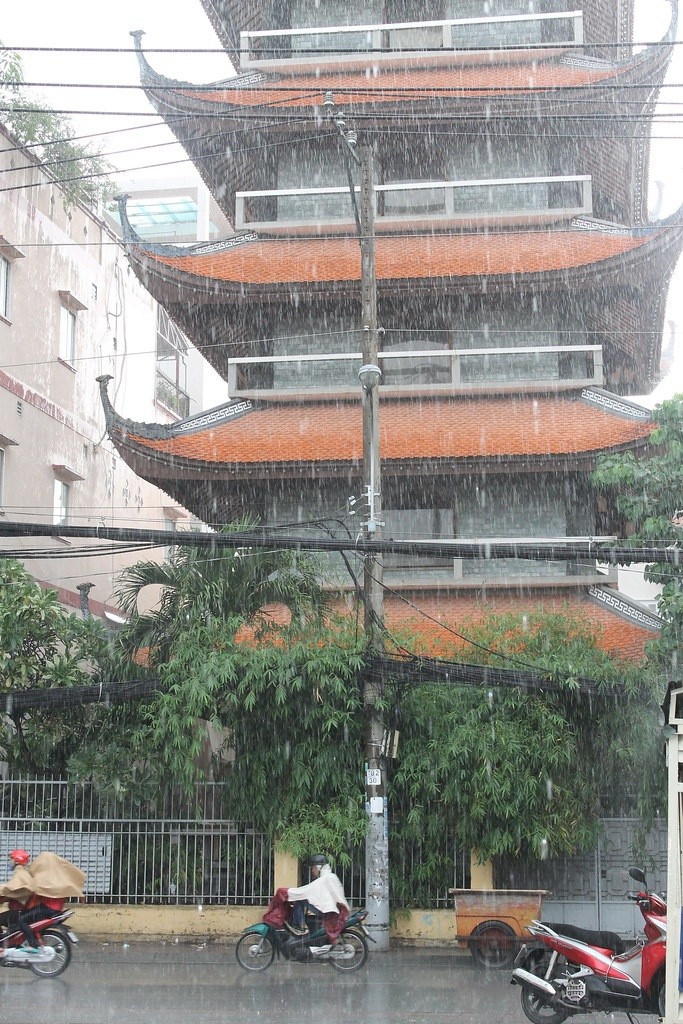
[294,926,309,933]
[0,951,4,958]
[20,946,39,954]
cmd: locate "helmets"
[11,850,29,865]
[309,855,327,865]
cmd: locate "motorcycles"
[508,866,666,1024]
[1,874,80,979]
[234,888,377,973]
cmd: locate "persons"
[278,853,351,935]
[0,848,65,959]
